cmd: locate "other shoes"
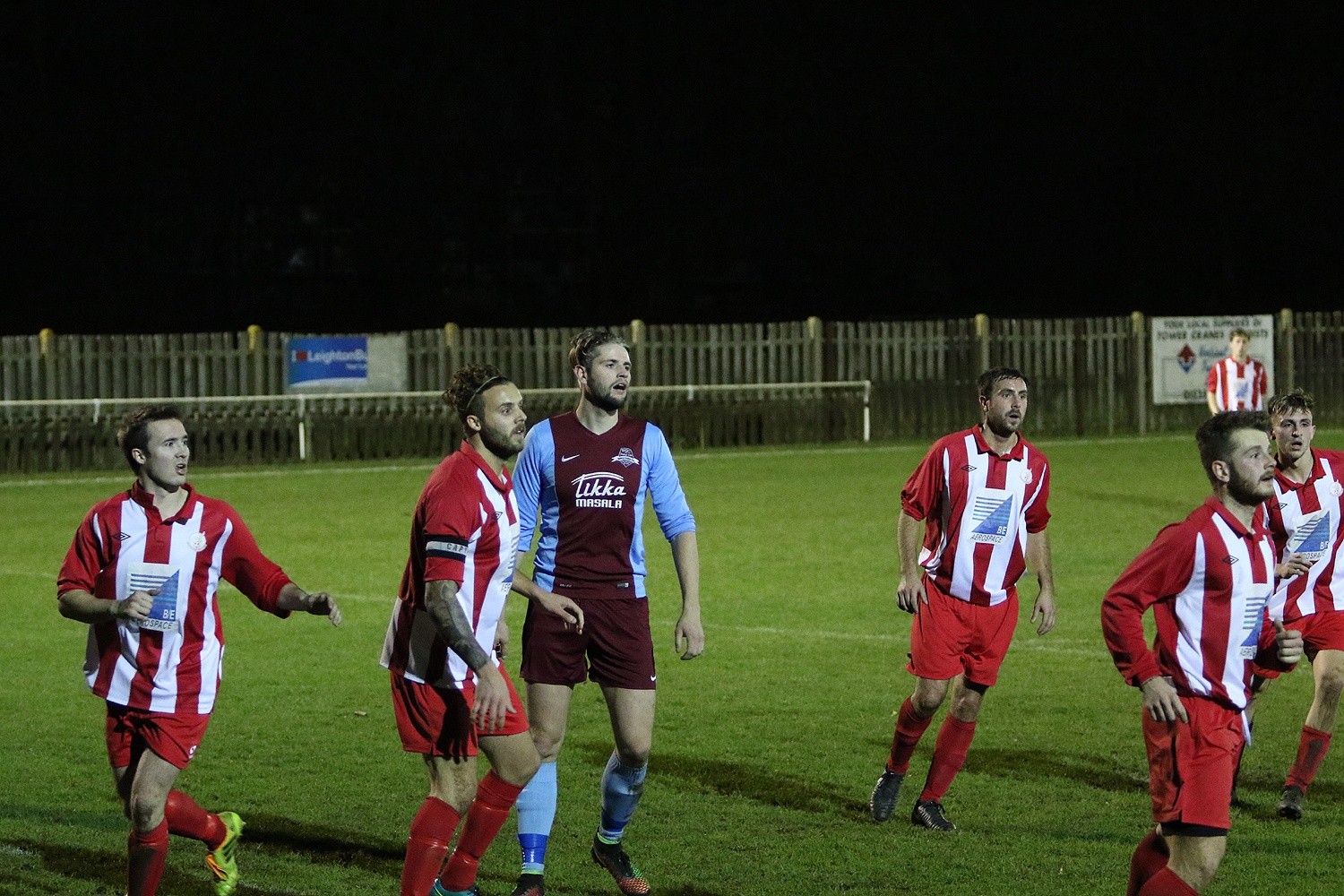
[429,879,476,896]
[1276,785,1303,821]
[1230,780,1239,805]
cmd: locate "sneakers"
[510,872,545,896]
[206,811,247,896]
[590,826,652,896]
[912,799,956,831]
[870,763,906,824]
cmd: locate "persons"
[493,330,703,896]
[57,405,344,896]
[869,367,1059,830]
[1098,328,1344,896]
[375,365,585,896]
